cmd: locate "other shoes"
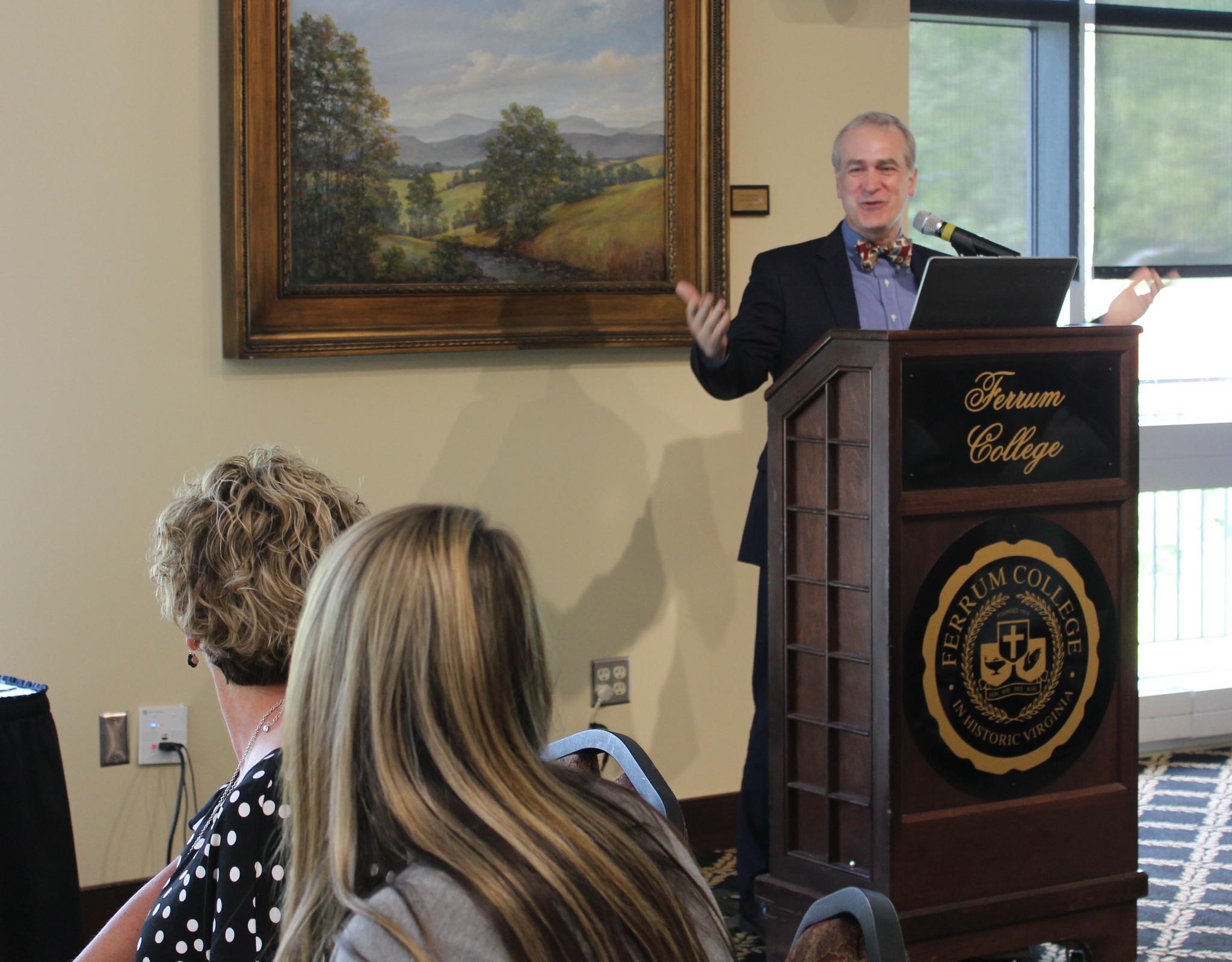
[736,893,764,938]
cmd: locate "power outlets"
[138,706,188,767]
[591,656,631,708]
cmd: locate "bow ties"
[854,237,912,273]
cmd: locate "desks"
[1,674,83,962]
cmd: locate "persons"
[59,442,372,961]
[253,501,743,962]
[674,112,1179,952]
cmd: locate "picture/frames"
[215,1,732,360]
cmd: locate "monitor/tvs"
[904,254,1080,330]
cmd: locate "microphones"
[912,211,1022,258]
